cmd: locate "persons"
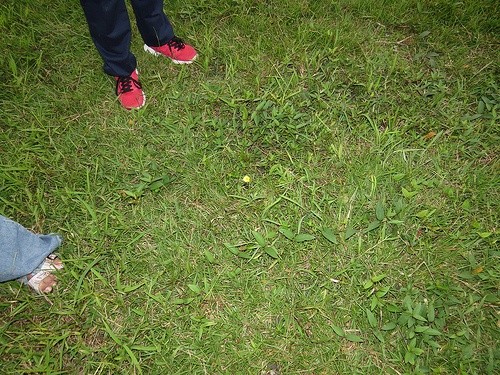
[1,204,67,306]
[79,0,198,112]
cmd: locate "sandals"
[19,253,64,297]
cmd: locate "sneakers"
[144,41,197,64]
[116,67,145,111]
